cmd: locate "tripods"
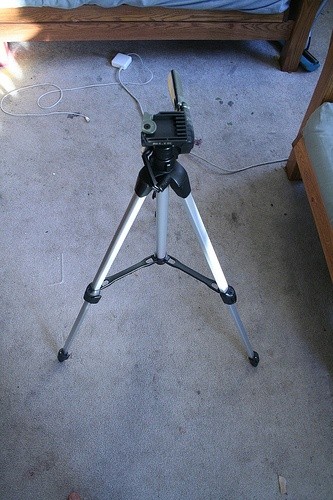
[57,70,259,368]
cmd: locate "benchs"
[286,29,333,284]
[0,0,326,71]
